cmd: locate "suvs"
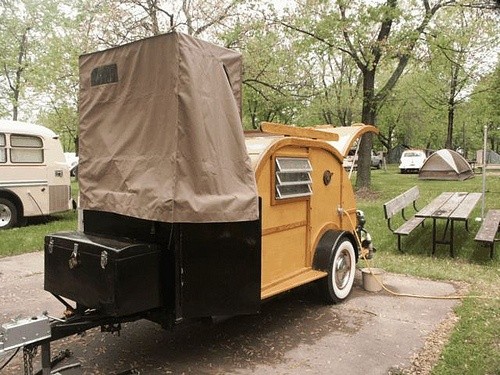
[347,147,383,169]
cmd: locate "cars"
[398,150,427,173]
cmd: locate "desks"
[415,191,483,257]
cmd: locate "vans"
[0,119,74,229]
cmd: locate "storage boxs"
[43,230,162,316]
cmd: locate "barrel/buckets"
[360,268,387,291]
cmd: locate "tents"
[418,148,475,182]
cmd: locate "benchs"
[383,184,429,251]
[474,207,500,260]
